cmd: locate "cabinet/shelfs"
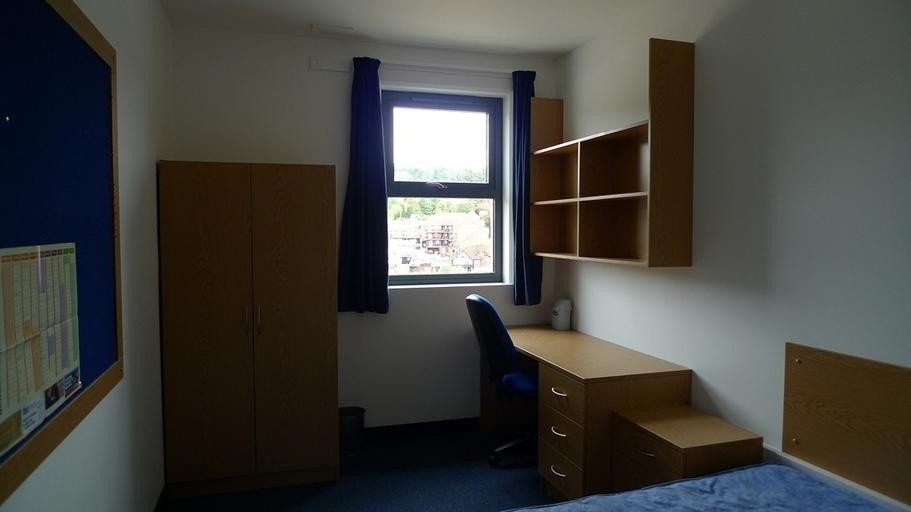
[156,157,345,494]
[525,37,696,272]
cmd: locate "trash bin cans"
[339,406,365,463]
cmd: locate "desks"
[507,325,693,496]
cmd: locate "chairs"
[465,293,538,465]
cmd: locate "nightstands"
[611,403,766,488]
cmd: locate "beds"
[509,463,895,511]
